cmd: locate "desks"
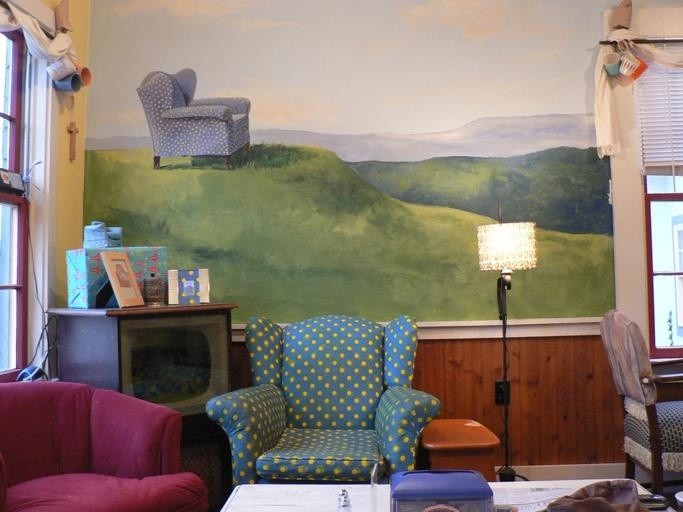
[421,418,501,479]
[221,478,674,512]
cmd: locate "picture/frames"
[100,251,146,308]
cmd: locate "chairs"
[205,315,441,483]
[600,310,683,495]
[1,378,208,512]
[136,68,250,170]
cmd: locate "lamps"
[477,222,539,480]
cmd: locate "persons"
[115,264,132,287]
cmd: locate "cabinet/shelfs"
[50,303,241,512]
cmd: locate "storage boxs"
[391,470,492,512]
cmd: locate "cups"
[47,53,91,92]
[603,51,648,80]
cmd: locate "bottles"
[144,273,162,307]
